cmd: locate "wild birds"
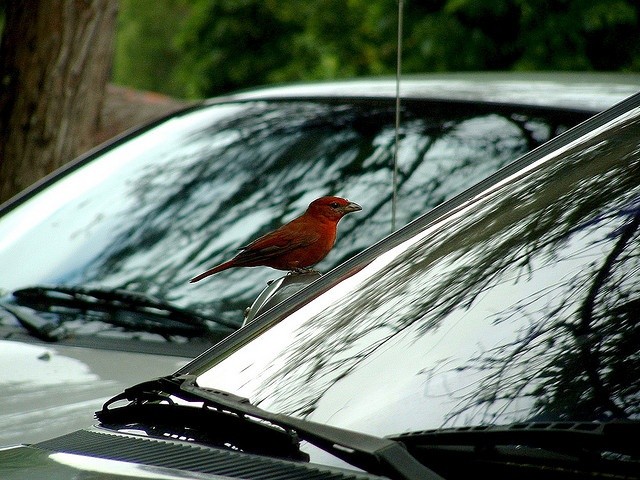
[190,195,362,284]
